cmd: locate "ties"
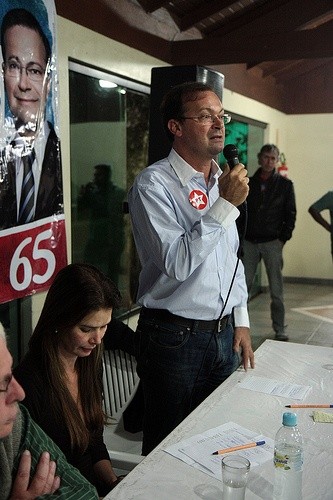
[17,146,36,225]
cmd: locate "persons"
[0,8,63,230]
[12,263,128,497]
[0,321,99,500]
[308,191,333,257]
[236,144,297,341]
[126,82,255,457]
[86,164,129,299]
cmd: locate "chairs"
[97,337,147,471]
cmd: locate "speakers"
[149,63,224,169]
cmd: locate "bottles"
[271,412,303,500]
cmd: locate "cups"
[221,455,251,500]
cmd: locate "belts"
[141,308,231,333]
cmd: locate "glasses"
[2,60,46,81]
[182,112,232,126]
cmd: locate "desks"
[99,339,333,500]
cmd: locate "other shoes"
[274,334,288,340]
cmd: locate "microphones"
[224,143,250,211]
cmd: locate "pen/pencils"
[285,404,333,409]
[212,440,266,456]
